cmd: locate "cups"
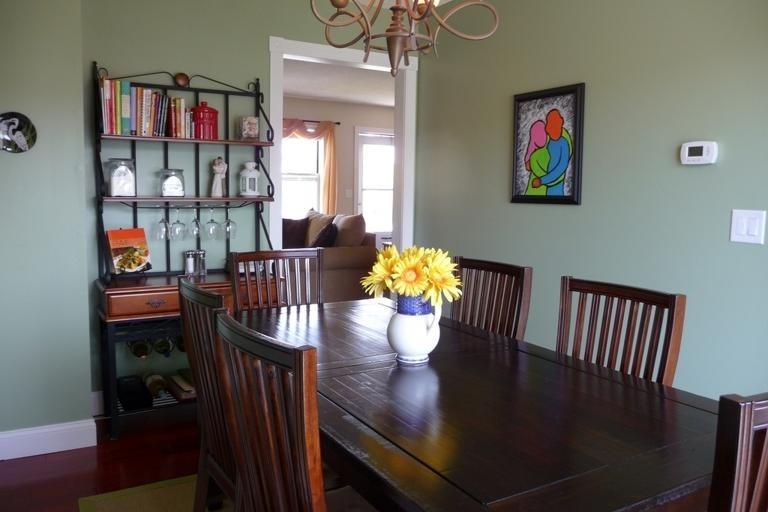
[238,116,259,139]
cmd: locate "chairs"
[706,392,768,512]
[177,273,348,512]
[450,256,533,342]
[214,313,378,512]
[227,247,324,314]
[555,275,687,387]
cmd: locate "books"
[100,74,195,139]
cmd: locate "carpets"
[78,464,381,512]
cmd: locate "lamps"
[310,0,500,78]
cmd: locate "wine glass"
[156,204,237,242]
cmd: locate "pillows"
[283,207,365,247]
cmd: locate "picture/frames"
[510,82,586,205]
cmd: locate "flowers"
[359,244,463,307]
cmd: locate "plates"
[118,251,149,272]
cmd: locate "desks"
[229,297,757,512]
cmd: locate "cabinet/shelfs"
[90,60,284,431]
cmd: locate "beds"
[283,232,379,306]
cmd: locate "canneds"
[102,156,136,196]
[184,250,206,280]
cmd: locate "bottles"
[154,337,171,359]
[142,369,166,399]
[107,158,136,197]
[175,334,187,353]
[131,337,151,359]
[190,101,219,140]
[185,250,207,278]
